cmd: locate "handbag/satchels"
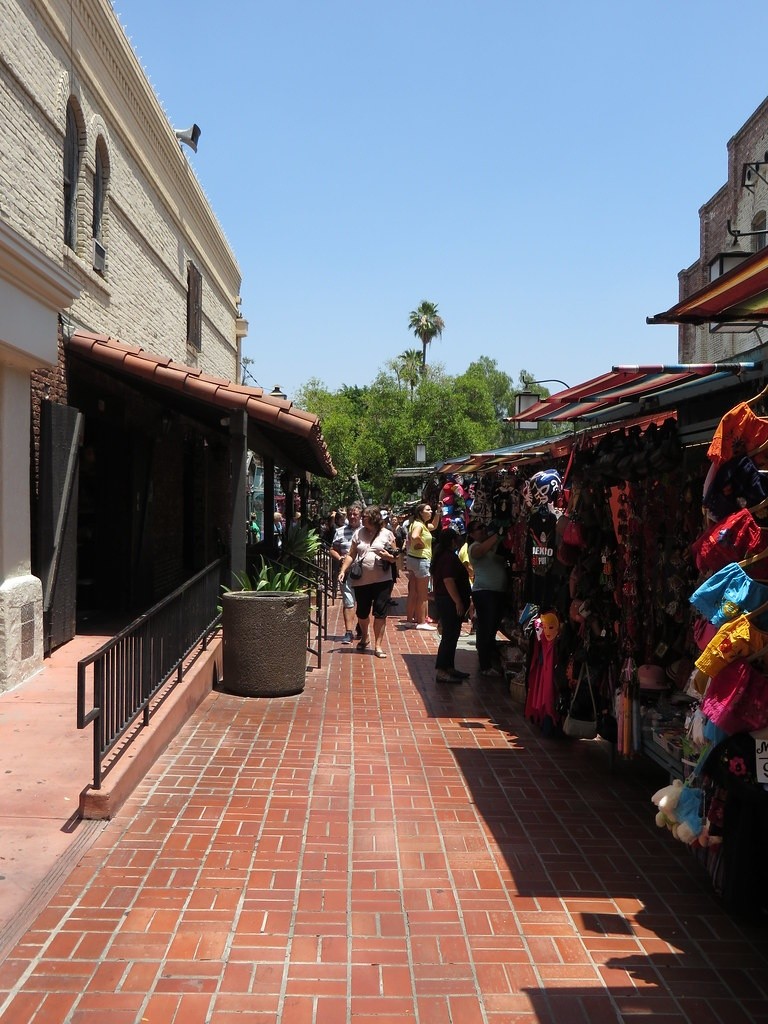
[556,484,616,742]
[494,564,513,596]
[350,561,362,579]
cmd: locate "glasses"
[471,523,486,531]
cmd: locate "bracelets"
[496,532,502,538]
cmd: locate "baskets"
[664,736,683,761]
[510,679,526,705]
[651,724,678,747]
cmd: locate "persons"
[428,528,473,685]
[406,501,443,631]
[337,504,400,658]
[272,500,421,606]
[467,519,509,678]
[407,506,433,624]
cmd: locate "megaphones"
[174,124,201,153]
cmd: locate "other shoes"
[448,668,470,678]
[435,671,462,683]
[483,668,504,678]
[459,631,469,637]
[416,623,438,631]
[404,621,412,628]
[426,616,433,623]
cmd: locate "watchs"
[435,510,441,515]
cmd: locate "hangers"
[710,382,768,782]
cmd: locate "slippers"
[357,641,371,649]
[387,598,399,606]
[374,652,387,658]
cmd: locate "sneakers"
[355,624,362,639]
[341,633,354,643]
